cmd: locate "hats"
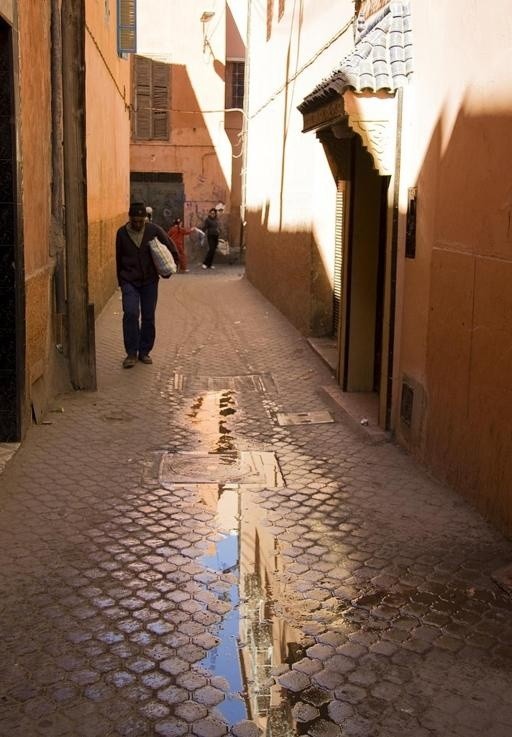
[129,203,148,217]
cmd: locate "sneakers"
[202,264,207,270]
[138,354,152,364]
[211,266,215,269]
[179,269,190,272]
[123,357,136,368]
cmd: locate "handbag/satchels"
[149,237,177,276]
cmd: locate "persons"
[197,208,223,269]
[114,200,182,369]
[169,217,197,273]
